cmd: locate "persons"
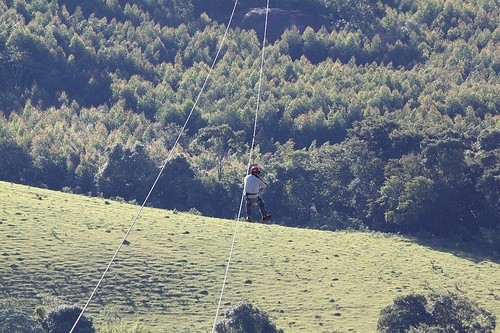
[242,166,271,222]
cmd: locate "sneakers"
[246,217,252,222]
[262,214,270,221]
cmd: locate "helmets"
[251,166,260,173]
